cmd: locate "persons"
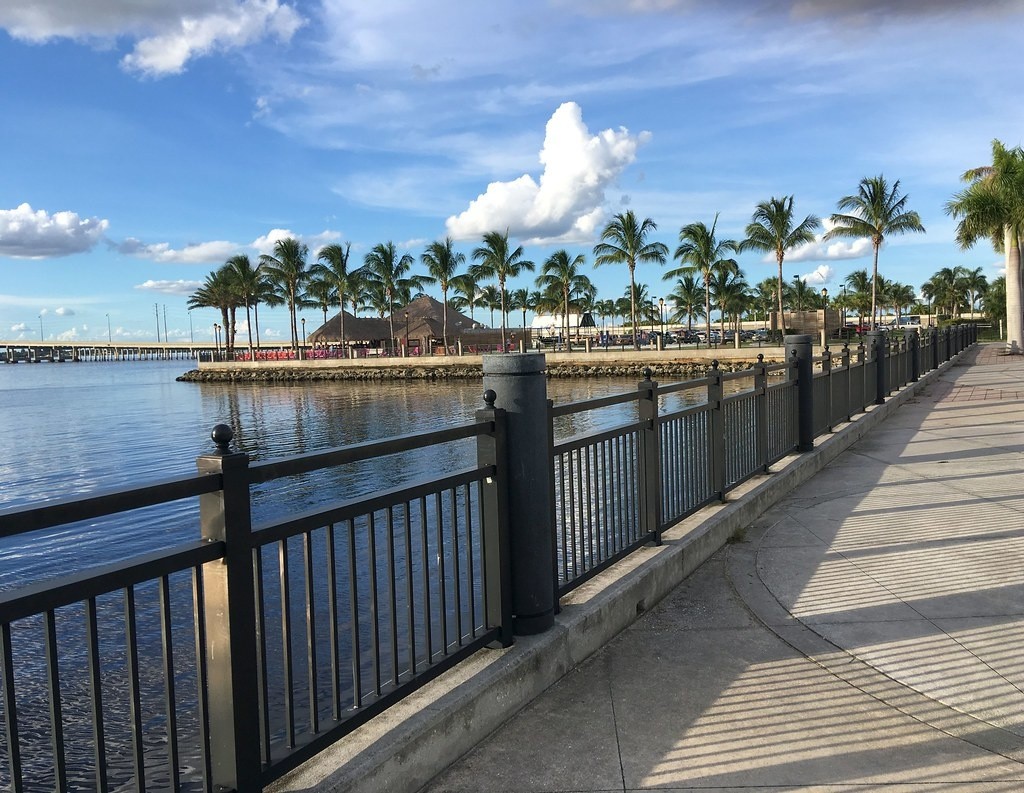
[642,332,646,346]
[316,344,341,350]
[595,333,600,343]
[506,337,512,346]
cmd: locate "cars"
[588,321,922,346]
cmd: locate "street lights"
[217,326,221,362]
[664,304,667,331]
[822,288,827,329]
[188,310,193,344]
[301,318,305,360]
[405,312,409,357]
[214,323,217,351]
[651,296,656,332]
[106,313,111,342]
[659,298,665,350]
[522,305,526,352]
[38,315,43,341]
[839,285,846,327]
[794,275,800,312]
